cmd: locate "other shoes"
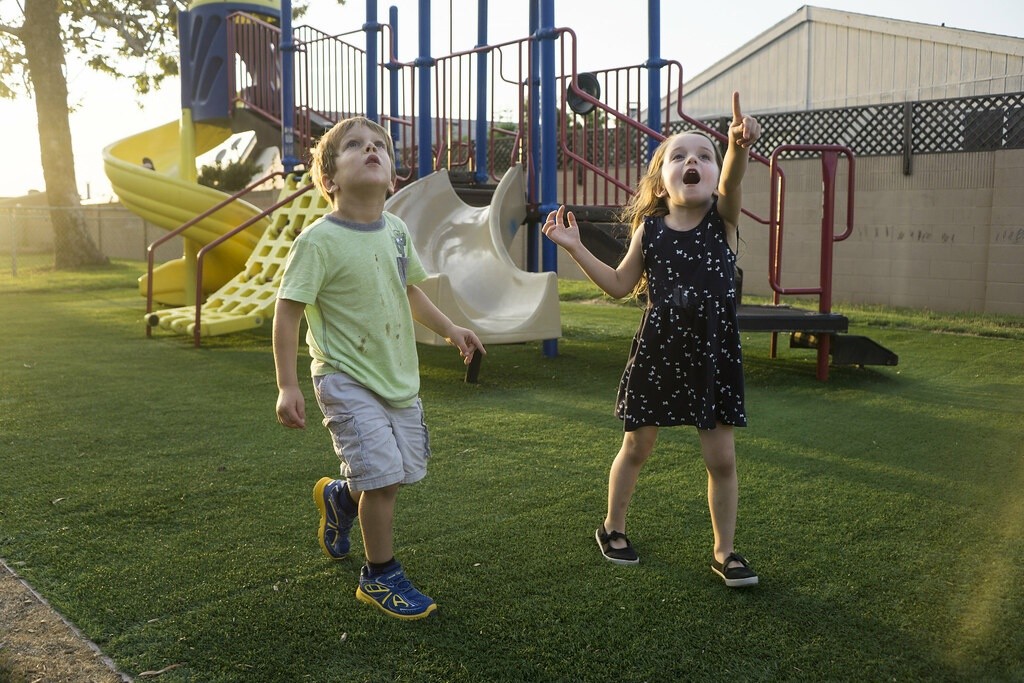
[711,553,759,586]
[595,524,639,564]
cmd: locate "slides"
[383,161,563,347]
[104,88,273,303]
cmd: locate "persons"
[268,114,486,623]
[543,89,764,587]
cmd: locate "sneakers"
[355,562,437,620]
[313,477,357,560]
